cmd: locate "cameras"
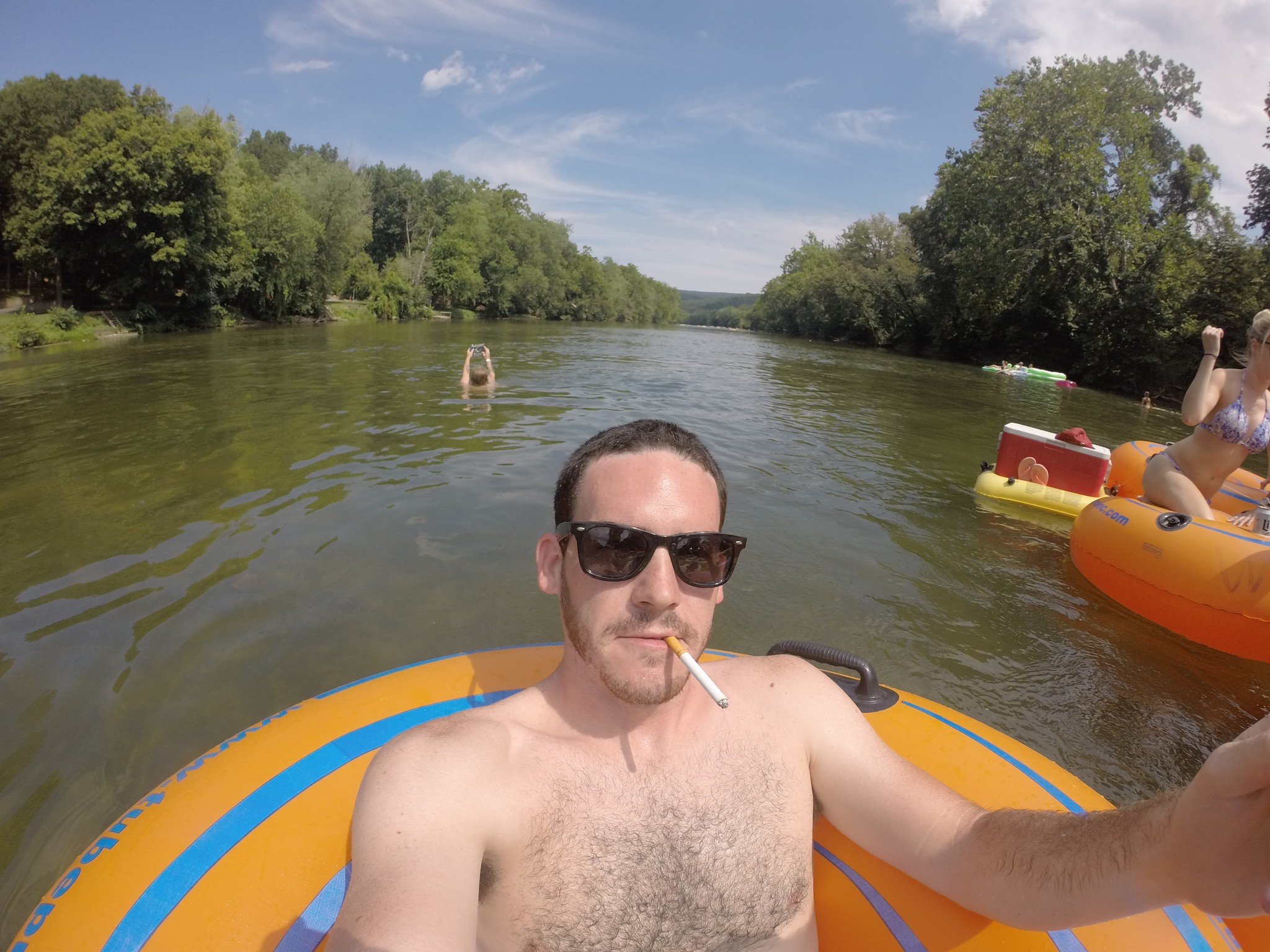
[470,343,485,353]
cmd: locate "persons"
[984,360,1032,370]
[1142,308,1270,528]
[461,346,495,386]
[1143,392,1157,408]
[324,419,1270,952]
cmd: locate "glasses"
[551,520,748,588]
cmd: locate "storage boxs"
[994,422,1111,497]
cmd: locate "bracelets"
[1203,353,1217,360]
[487,359,491,362]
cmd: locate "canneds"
[1252,505,1270,537]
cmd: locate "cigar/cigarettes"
[662,636,729,708]
